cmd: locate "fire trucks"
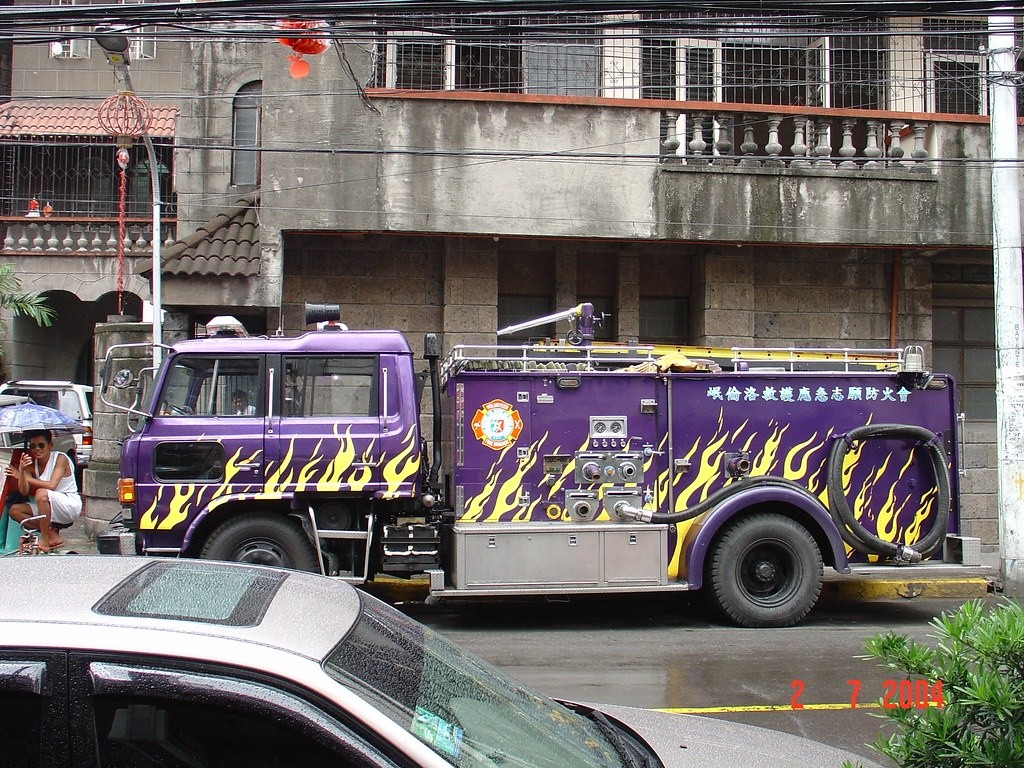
[88,299,1005,627]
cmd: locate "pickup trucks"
[0,379,93,479]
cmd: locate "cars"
[0,544,885,768]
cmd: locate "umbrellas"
[0,402,83,453]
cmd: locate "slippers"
[49,541,65,550]
[24,546,48,554]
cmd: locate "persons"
[231,390,256,416]
[5,430,82,548]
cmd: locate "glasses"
[28,442,49,449]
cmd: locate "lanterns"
[276,16,332,54]
